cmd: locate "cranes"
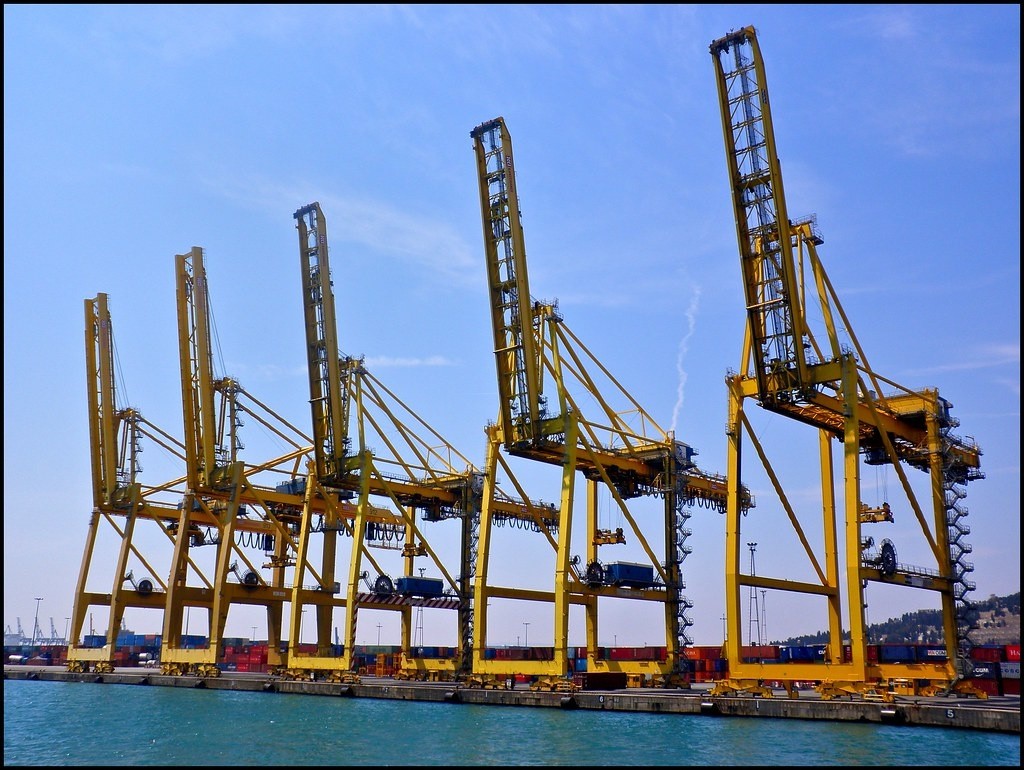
[17,617,26,637]
[35,617,44,637]
[50,617,59,637]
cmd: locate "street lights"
[65,617,71,638]
[301,611,307,643]
[253,627,258,641]
[32,598,44,646]
[377,626,383,646]
[523,622,531,646]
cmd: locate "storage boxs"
[604,561,653,586]
[4,645,84,665]
[84,631,1020,697]
[191,534,204,546]
[276,478,353,502]
[177,497,247,516]
[396,576,444,597]
